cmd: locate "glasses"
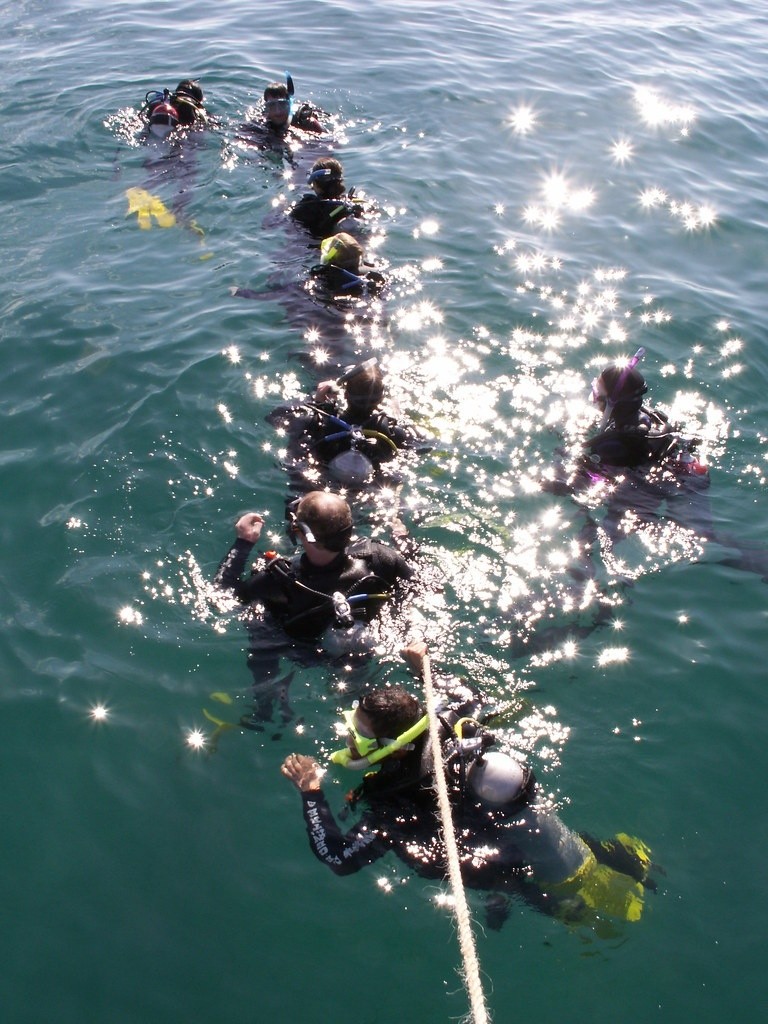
[262,99,290,114]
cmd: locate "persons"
[280,650,578,922]
[148,78,205,136]
[261,157,364,232]
[265,82,291,126]
[266,365,409,452]
[230,233,385,334]
[207,490,414,731]
[542,362,766,642]
